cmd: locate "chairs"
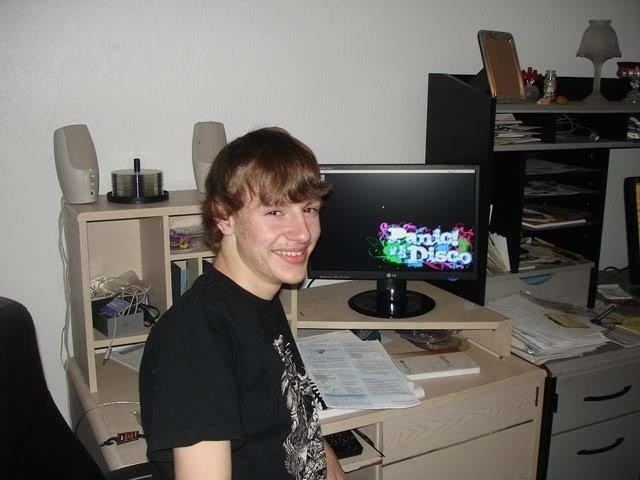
[0,297,108,479]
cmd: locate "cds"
[111,169,164,198]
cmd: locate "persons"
[139,127,350,480]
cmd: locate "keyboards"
[322,430,363,460]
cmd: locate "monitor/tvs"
[308,164,487,318]
[619,176,640,297]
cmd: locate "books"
[393,352,480,381]
[521,204,588,230]
[298,330,425,419]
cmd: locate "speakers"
[192,122,227,193]
[54,124,99,204]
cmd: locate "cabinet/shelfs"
[425,70,640,309]
[544,346,639,479]
[63,357,548,480]
[63,189,296,395]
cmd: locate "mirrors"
[477,29,526,103]
